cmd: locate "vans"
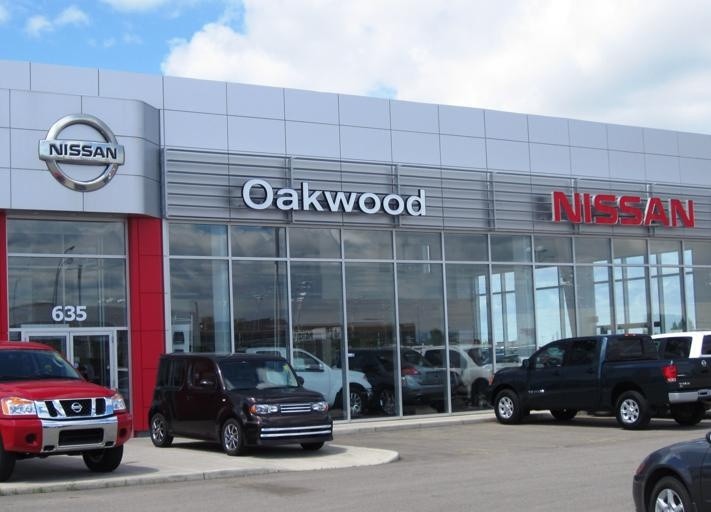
[649,330,711,359]
[146,351,334,457]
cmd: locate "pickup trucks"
[491,333,709,430]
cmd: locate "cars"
[633,430,711,512]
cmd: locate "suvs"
[70,354,128,412]
[0,339,131,483]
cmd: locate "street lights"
[48,256,73,322]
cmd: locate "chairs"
[246,366,269,387]
